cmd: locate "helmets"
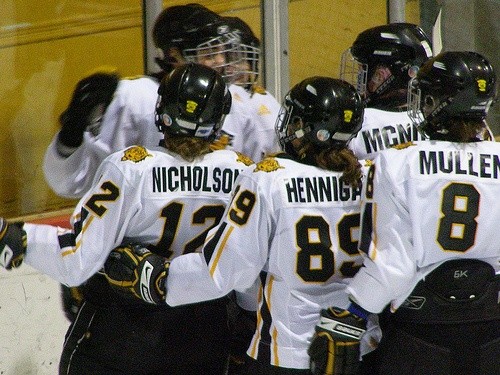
[154,62,232,142]
[152,3,261,85]
[340,23,433,108]
[407,51,497,139]
[274,76,364,155]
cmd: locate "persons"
[0,3,500,375]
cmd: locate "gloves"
[85,236,170,306]
[58,72,118,148]
[307,306,369,375]
[0,217,27,270]
